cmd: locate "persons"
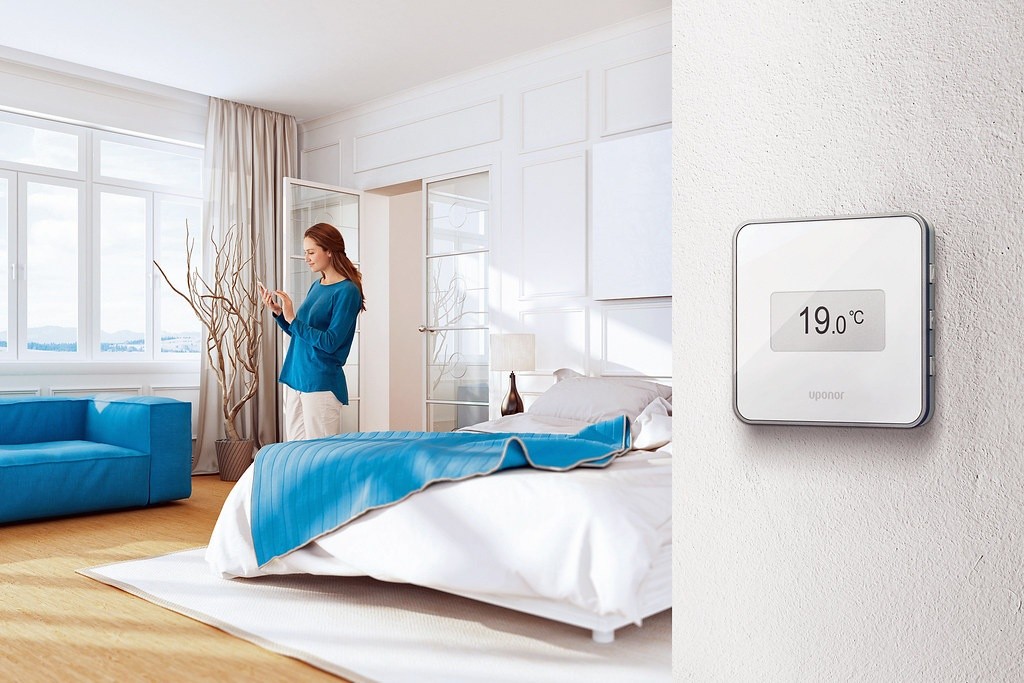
[260,223,367,442]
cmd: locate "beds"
[204,368,672,643]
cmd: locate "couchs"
[0,393,192,524]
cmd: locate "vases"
[214,439,254,481]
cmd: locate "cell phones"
[257,282,275,305]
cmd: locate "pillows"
[526,369,672,425]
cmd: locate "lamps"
[489,333,534,417]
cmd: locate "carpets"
[75,545,671,683]
